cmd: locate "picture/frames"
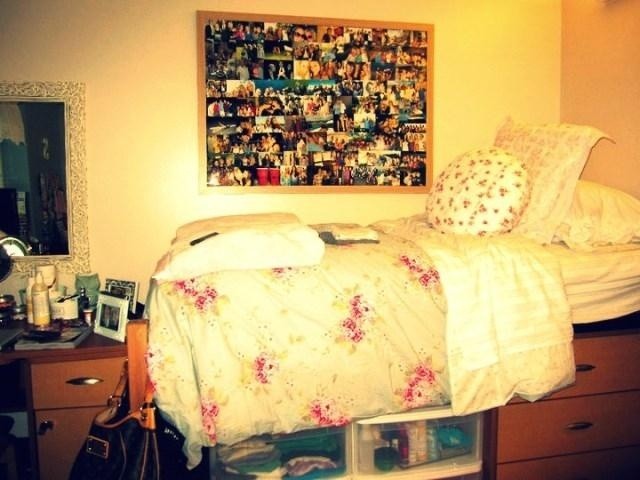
[105,278,139,315]
[196,10,434,194]
[94,293,129,343]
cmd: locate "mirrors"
[0,80,92,275]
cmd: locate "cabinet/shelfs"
[484,328,640,480]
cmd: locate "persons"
[206,21,427,184]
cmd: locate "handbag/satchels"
[68,404,161,479]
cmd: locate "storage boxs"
[209,404,484,480]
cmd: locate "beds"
[143,212,640,469]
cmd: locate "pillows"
[545,179,640,249]
[426,147,531,237]
[493,115,615,246]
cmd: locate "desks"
[0,318,149,480]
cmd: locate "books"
[14,327,92,351]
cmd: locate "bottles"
[11,308,24,327]
[78,287,90,323]
[25,277,50,327]
